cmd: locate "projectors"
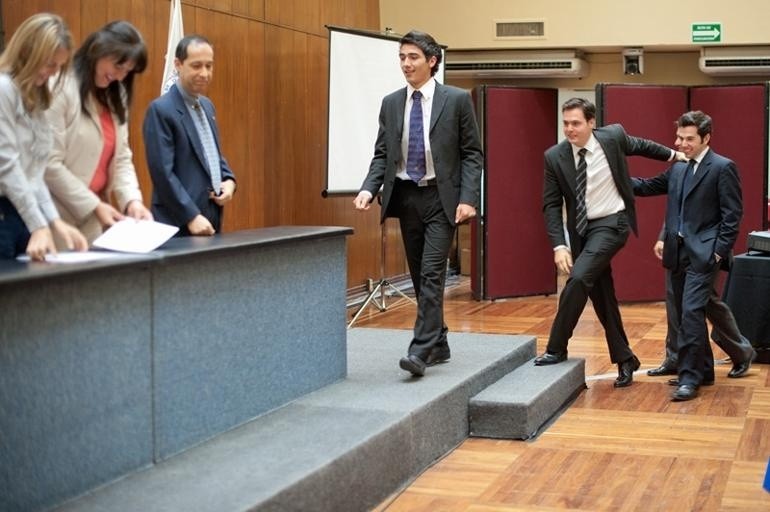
[746,230,770,251]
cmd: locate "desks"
[712,252,769,366]
[0,225,353,510]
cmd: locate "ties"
[574,149,588,237]
[195,100,221,196]
[678,159,696,235]
[406,91,427,183]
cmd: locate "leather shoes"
[614,356,641,387]
[398,354,425,377]
[426,352,450,366]
[534,350,567,364]
[671,384,698,400]
[669,376,715,386]
[648,361,677,376]
[728,350,757,376]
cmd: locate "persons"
[646,121,757,378]
[0,14,89,261]
[352,31,483,376]
[533,98,690,385]
[628,111,742,400]
[44,20,154,253]
[142,35,237,236]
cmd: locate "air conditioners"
[698,47,770,74]
[444,51,589,79]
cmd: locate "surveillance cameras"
[622,47,643,75]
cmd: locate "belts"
[404,180,436,187]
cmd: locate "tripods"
[347,222,418,332]
